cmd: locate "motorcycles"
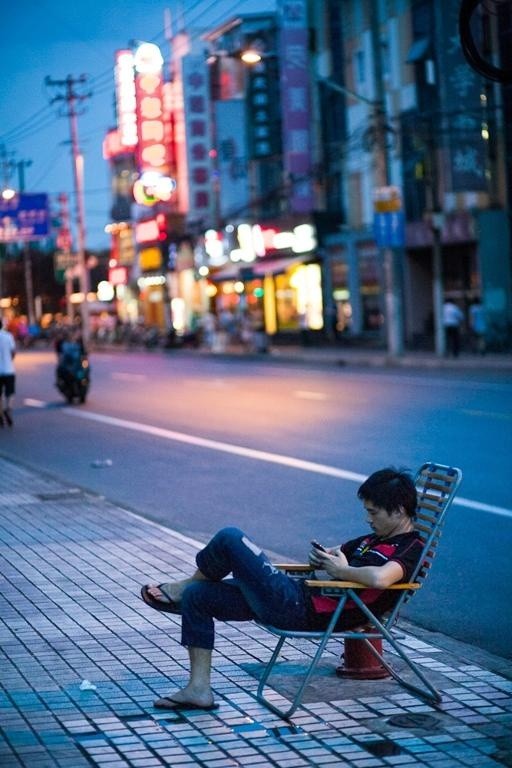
[54,349,90,405]
[59,319,206,352]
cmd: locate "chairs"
[252,460,464,720]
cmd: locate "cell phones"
[309,539,328,553]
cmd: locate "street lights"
[232,45,409,359]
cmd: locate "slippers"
[153,696,220,711]
[140,582,183,616]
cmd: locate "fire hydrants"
[333,626,389,682]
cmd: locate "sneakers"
[0,408,14,428]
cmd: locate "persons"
[0,321,20,428]
[138,465,424,713]
[56,325,85,378]
[442,295,465,360]
[467,297,494,357]
